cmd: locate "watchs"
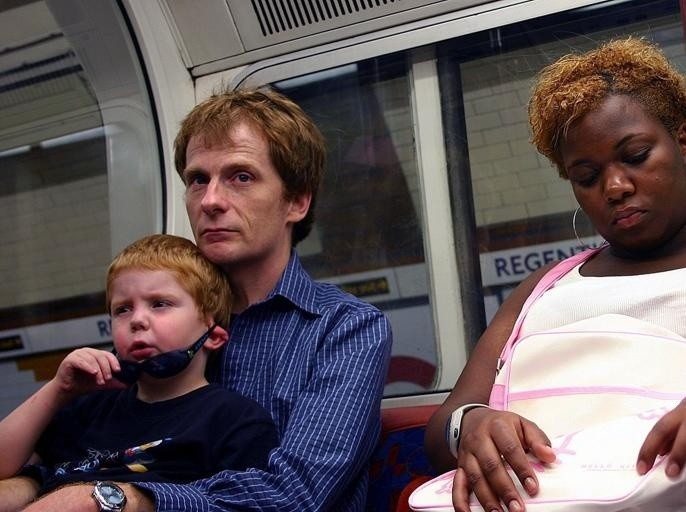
[442,401,492,464]
[90,476,129,512]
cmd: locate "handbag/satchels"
[408,314,686,511]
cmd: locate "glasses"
[110,324,217,384]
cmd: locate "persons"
[0,231,279,512]
[0,77,395,512]
[395,35,685,512]
